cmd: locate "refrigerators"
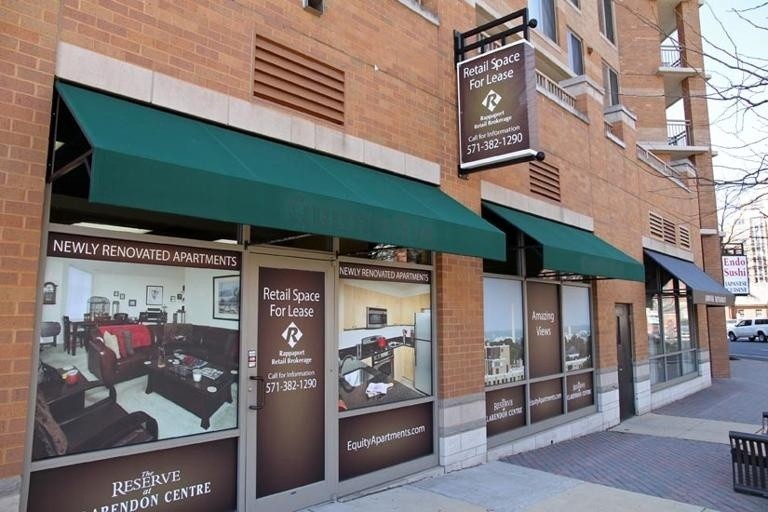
[414,310,432,396]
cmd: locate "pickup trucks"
[727,318,767,342]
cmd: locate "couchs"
[86,322,239,390]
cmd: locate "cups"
[68,370,81,384]
[168,358,180,364]
[192,368,203,383]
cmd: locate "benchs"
[728,409,768,499]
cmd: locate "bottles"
[158,347,166,368]
[761,410,768,434]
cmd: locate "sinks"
[342,366,377,388]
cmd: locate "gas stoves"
[372,348,393,361]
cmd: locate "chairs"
[62,312,129,357]
[31,381,159,461]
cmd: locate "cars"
[646,334,681,366]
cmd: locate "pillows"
[96,323,224,360]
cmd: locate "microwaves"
[365,306,388,329]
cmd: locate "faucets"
[338,354,355,374]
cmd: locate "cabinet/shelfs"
[343,283,430,332]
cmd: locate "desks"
[38,364,89,417]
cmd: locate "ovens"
[374,358,393,379]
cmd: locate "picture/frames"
[212,274,240,321]
[112,284,164,307]
